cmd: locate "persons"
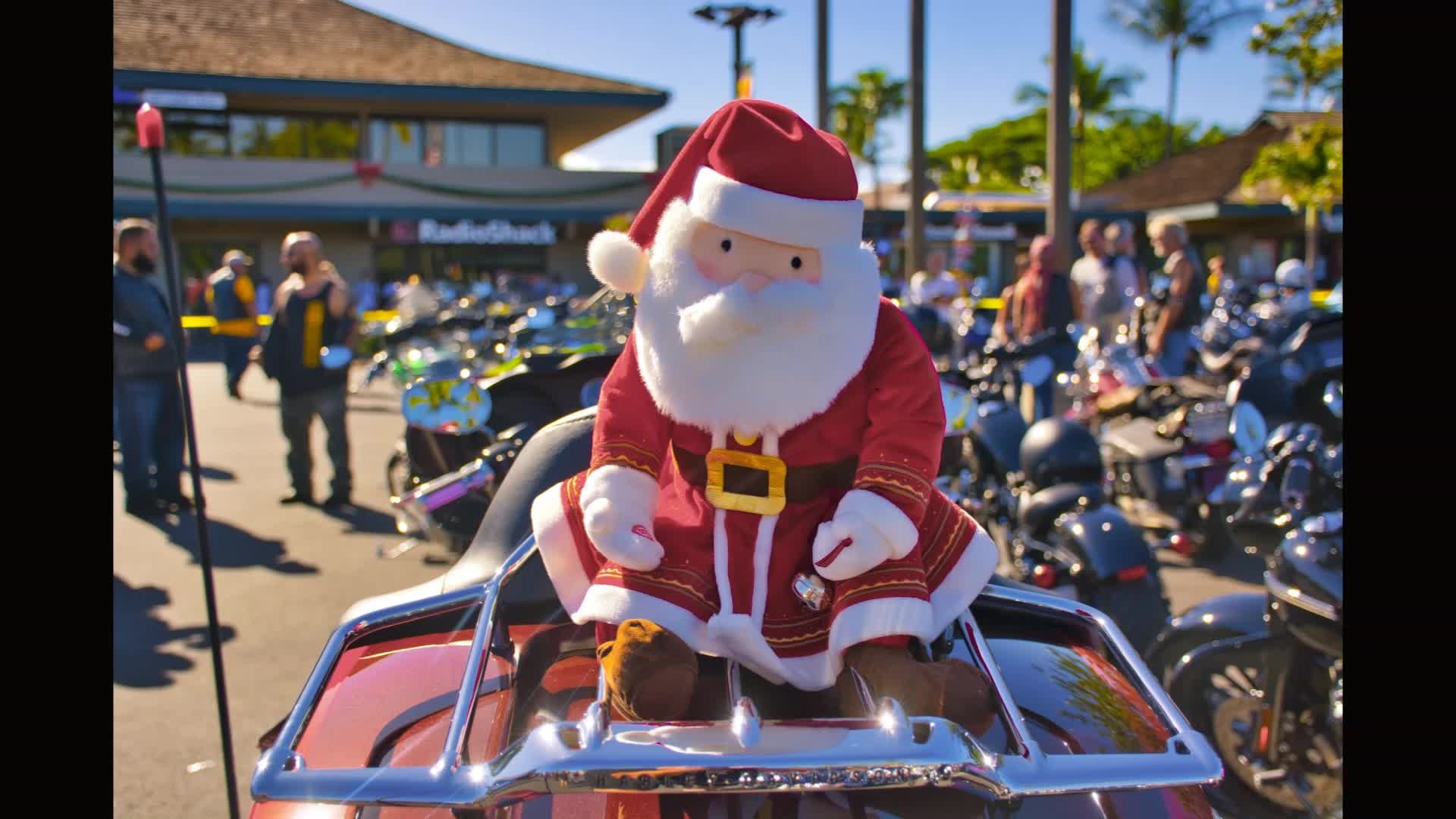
[206,250,261,400]
[910,216,1227,421]
[113,217,190,519]
[257,231,354,511]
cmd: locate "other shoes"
[324,495,350,508]
[126,498,168,516]
[283,494,313,504]
[154,492,192,513]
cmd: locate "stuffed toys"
[529,100,999,739]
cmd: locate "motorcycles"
[931,273,1341,819]
[366,329,638,556]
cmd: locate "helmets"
[973,400,1024,471]
[1020,416,1103,486]
